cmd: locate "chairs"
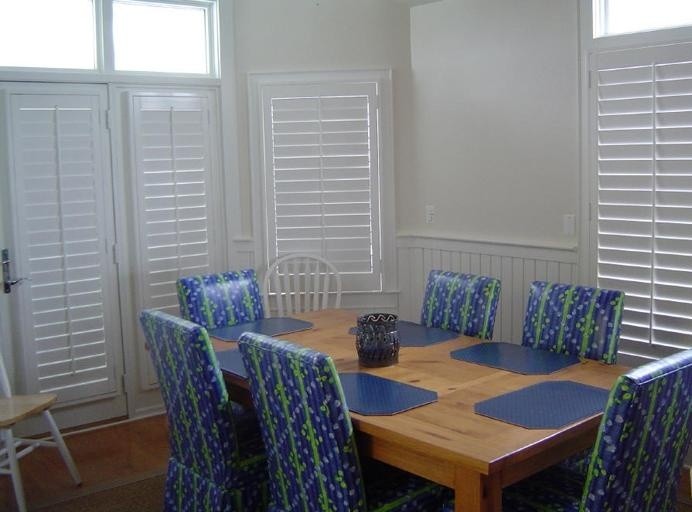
[419,268,502,341]
[519,279,627,366]
[262,251,343,317]
[137,308,270,511]
[175,267,267,330]
[1,348,86,510]
[235,330,456,511]
[577,345,692,510]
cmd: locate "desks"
[197,304,642,512]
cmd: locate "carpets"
[29,465,171,511]
